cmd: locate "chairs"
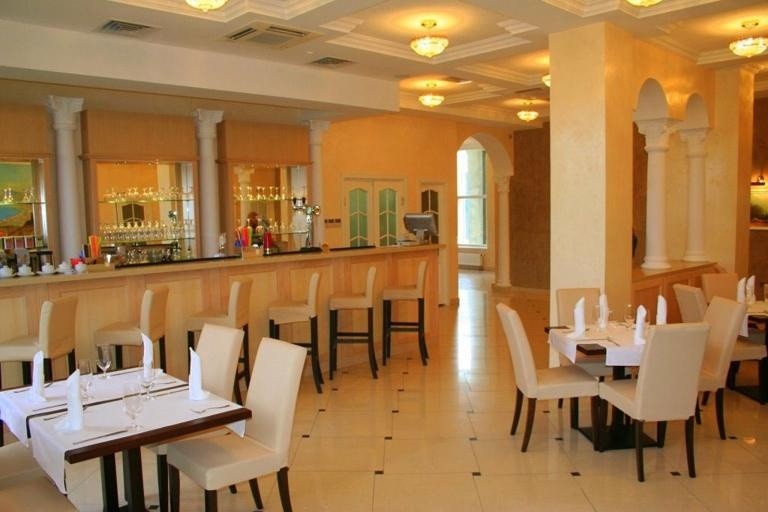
[0,437,85,511]
[168,332,308,510]
[155,320,248,509]
[496,273,768,483]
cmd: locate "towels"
[27,350,44,404]
[60,368,85,429]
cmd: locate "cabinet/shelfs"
[215,117,313,257]
[77,107,201,265]
[0,104,61,270]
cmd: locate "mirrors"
[94,157,196,259]
[231,162,307,253]
[0,158,47,254]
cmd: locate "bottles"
[216,215,280,257]
[1,262,88,276]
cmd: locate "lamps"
[629,1,661,7]
[418,83,444,108]
[183,0,228,12]
[543,75,552,86]
[410,21,450,60]
[728,22,768,58]
[515,103,539,122]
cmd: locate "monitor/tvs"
[404,213,438,243]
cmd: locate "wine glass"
[592,304,637,340]
[99,185,181,242]
[233,184,295,201]
[2,187,38,203]
[73,354,158,431]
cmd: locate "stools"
[0,258,435,396]
